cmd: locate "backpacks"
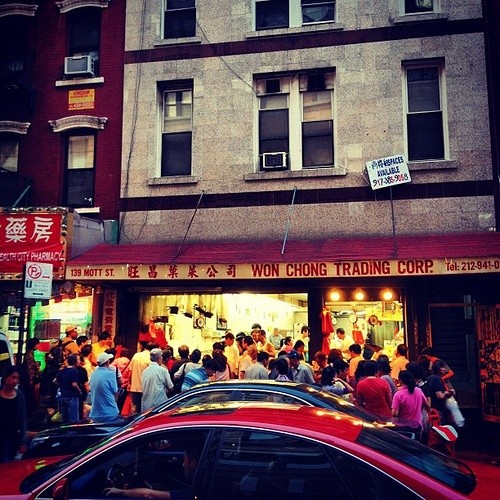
[45,340,73,368]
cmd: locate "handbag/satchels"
[446,396,464,428]
[167,363,186,399]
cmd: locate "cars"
[0,374,500,500]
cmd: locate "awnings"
[66,232,500,279]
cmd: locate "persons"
[0,324,464,450]
[104,447,202,500]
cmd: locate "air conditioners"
[263,152,287,169]
[64,55,96,78]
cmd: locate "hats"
[150,348,162,360]
[286,350,298,357]
[97,352,114,365]
[65,326,78,332]
